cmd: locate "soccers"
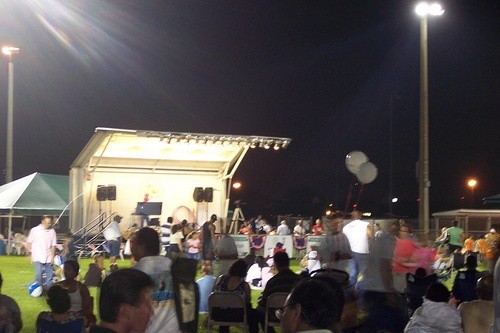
[28,281,43,297]
[54,254,64,267]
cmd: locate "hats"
[113,215,122,222]
[211,214,217,220]
[426,282,450,302]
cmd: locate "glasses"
[274,300,295,320]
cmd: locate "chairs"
[206,292,249,333]
[264,293,289,333]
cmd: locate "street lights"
[1,45,20,183]
[415,2,445,236]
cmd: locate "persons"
[27,215,57,295]
[103,215,123,273]
[0,210,500,333]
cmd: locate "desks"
[229,232,295,259]
[307,236,323,255]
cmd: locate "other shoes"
[109,263,118,272]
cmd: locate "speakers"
[204,188,213,202]
[107,185,116,199]
[97,185,108,200]
[193,187,204,202]
